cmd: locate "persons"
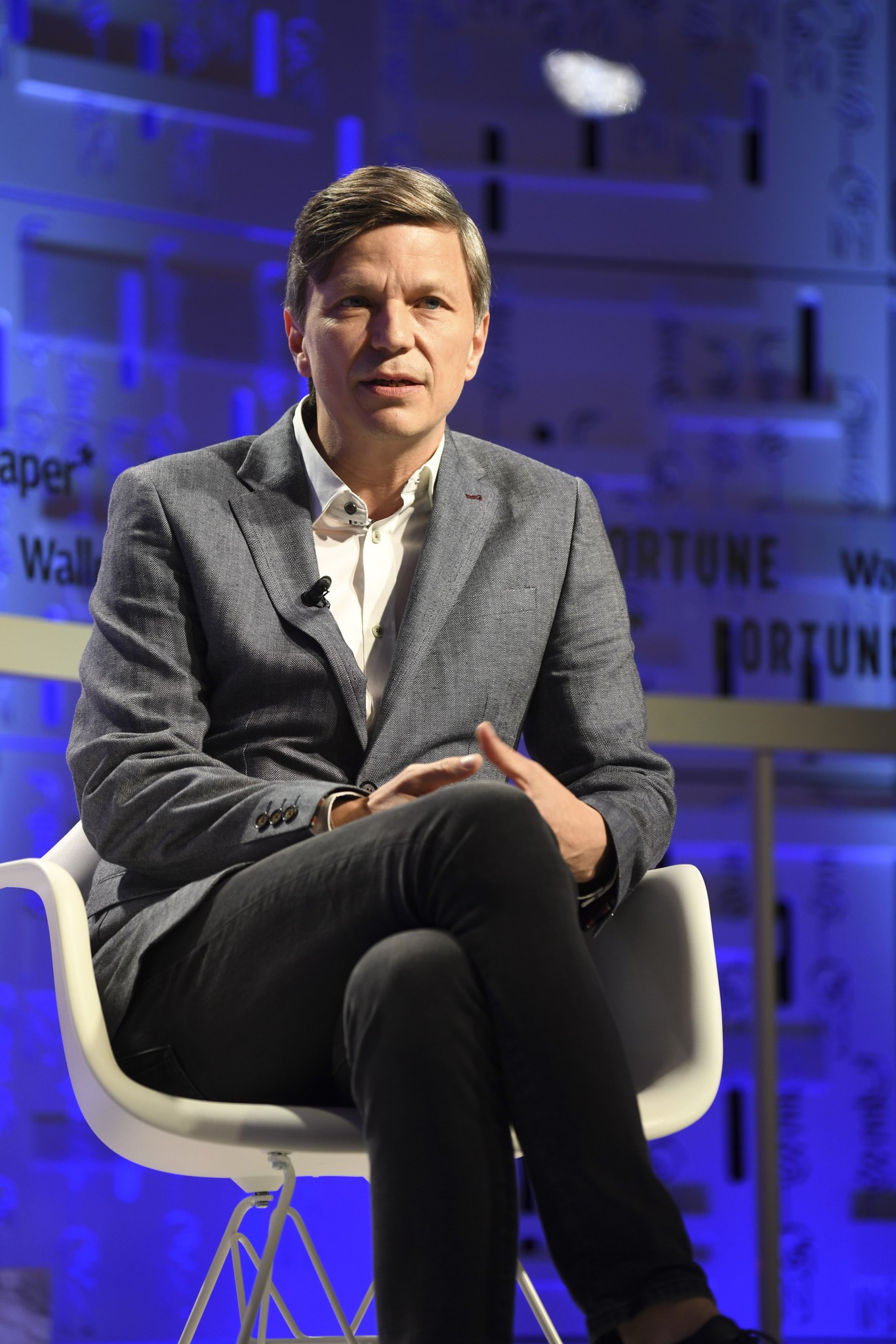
[67,164,772,1343]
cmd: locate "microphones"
[300,575,333,610]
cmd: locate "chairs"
[0,819,725,1344]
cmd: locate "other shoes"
[593,1313,773,1344]
